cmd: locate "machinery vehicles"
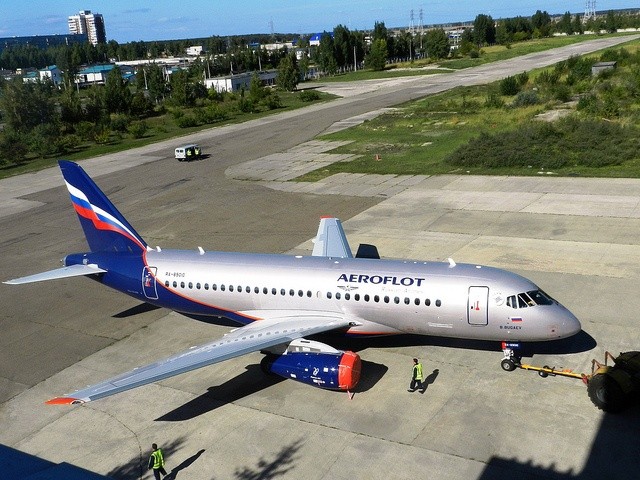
[513,348,640,414]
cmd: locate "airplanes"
[1,158,582,406]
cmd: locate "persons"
[194,147,200,160]
[407,358,423,392]
[186,148,192,162]
[147,442,167,480]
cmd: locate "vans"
[175,144,201,161]
